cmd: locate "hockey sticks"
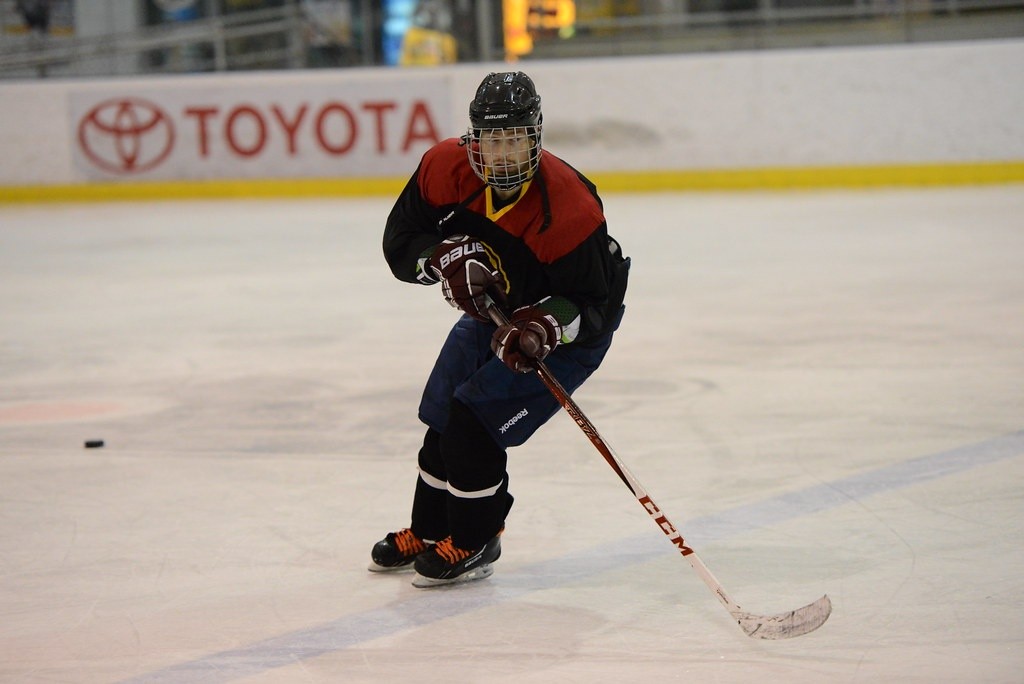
[484,297,832,644]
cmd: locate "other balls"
[83,437,106,453]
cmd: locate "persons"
[368,72,631,589]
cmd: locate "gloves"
[490,305,563,374]
[429,233,510,322]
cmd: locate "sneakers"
[411,522,505,588]
[367,528,435,572]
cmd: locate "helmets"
[457,71,543,191]
